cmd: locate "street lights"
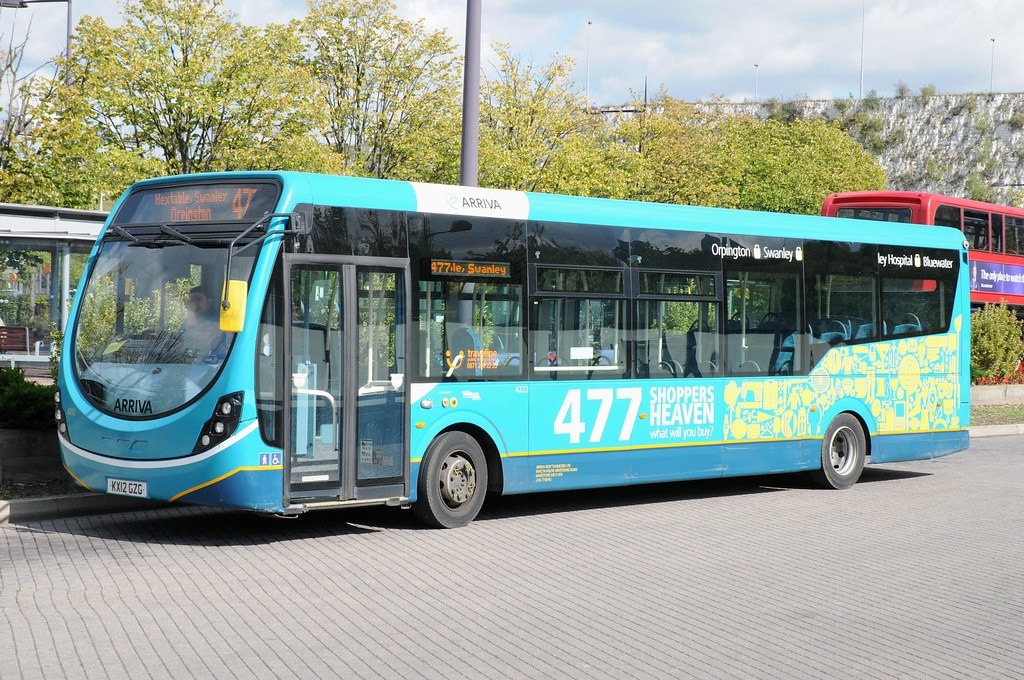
[0,0,71,191]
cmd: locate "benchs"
[0,326,34,354]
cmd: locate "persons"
[175,287,225,364]
[970,264,979,290]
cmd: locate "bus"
[820,192,1024,386]
[55,172,971,531]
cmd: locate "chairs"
[443,311,921,379]
